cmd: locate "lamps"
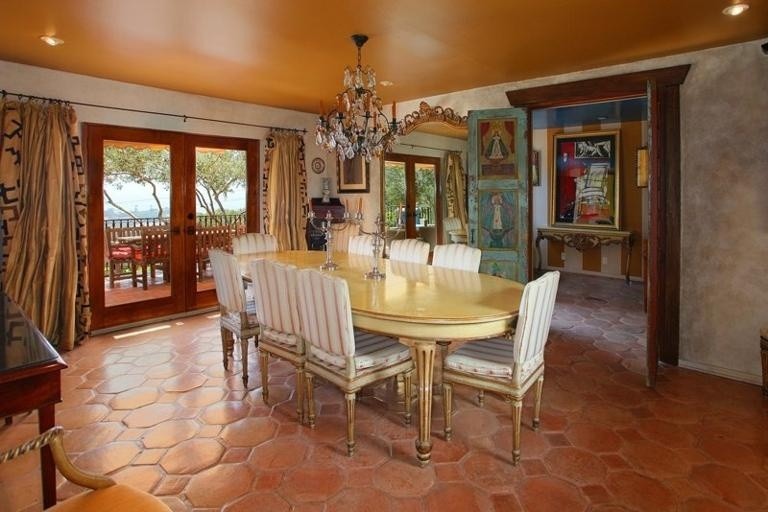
[314,34,402,163]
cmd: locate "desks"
[535,226,633,286]
[0,291,68,511]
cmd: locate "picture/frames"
[477,117,519,180]
[336,150,370,194]
[636,146,650,189]
[477,188,520,251]
[550,129,622,231]
[478,259,519,281]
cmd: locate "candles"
[345,200,349,212]
[398,204,402,224]
[309,201,312,212]
[358,199,362,209]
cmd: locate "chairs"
[106,222,247,290]
[441,270,560,468]
[295,269,417,458]
[249,257,362,425]
[348,226,482,273]
[0,425,174,512]
[231,232,279,255]
[207,248,274,389]
